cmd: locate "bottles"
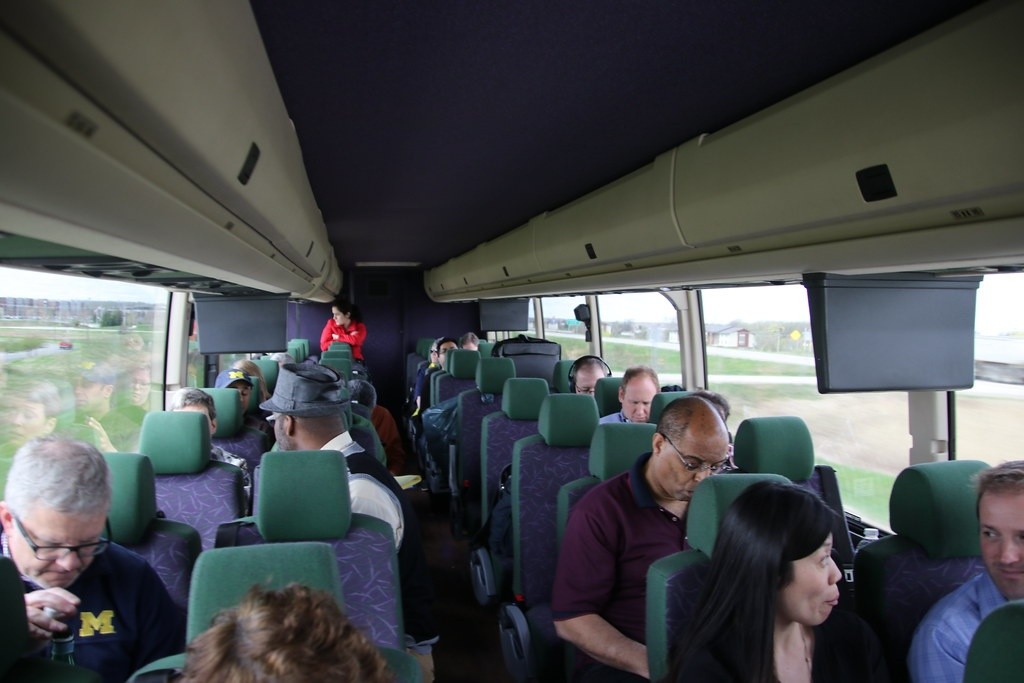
[50,627,77,665]
[855,528,879,553]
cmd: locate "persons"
[320,300,367,366]
[259,363,440,683]
[666,481,891,683]
[907,460,1024,683]
[171,387,252,516]
[179,582,396,683]
[215,359,272,434]
[0,330,151,452]
[460,332,479,351]
[413,336,459,491]
[0,433,187,683]
[551,397,729,683]
[568,355,612,396]
[599,365,661,425]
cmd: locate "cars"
[57,340,73,349]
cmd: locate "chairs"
[96,336,1024,683]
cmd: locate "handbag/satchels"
[471,463,513,558]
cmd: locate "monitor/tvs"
[803,271,984,394]
[194,293,290,355]
[477,298,529,331]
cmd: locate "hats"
[259,363,350,418]
[214,368,254,388]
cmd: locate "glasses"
[265,413,296,428]
[661,433,728,475]
[574,384,595,395]
[11,508,112,560]
[430,349,438,353]
